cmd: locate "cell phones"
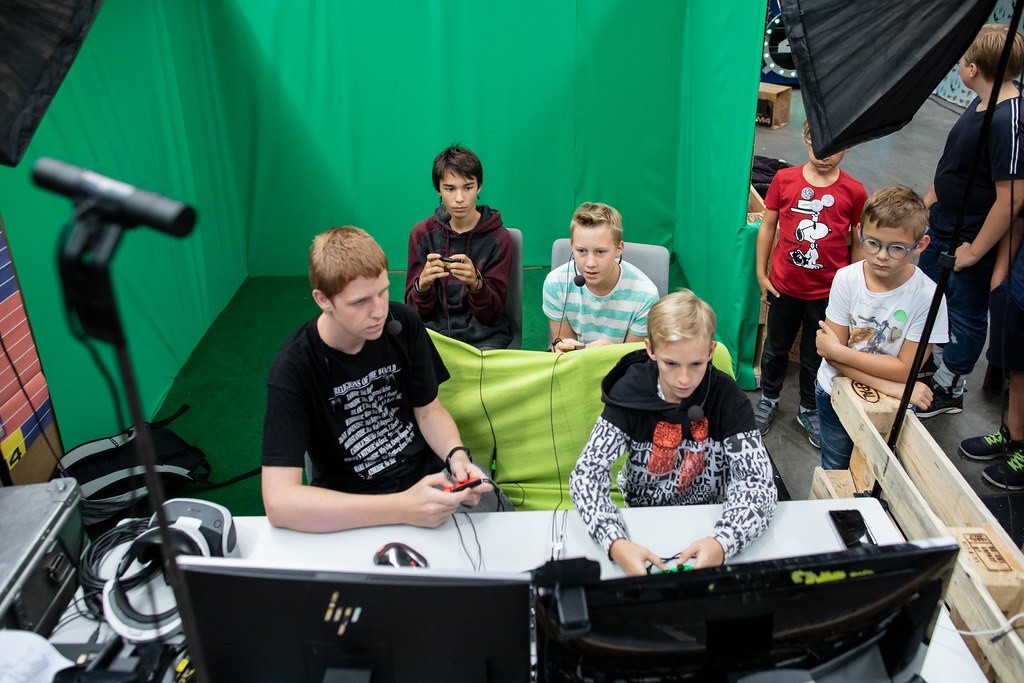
[828,509,878,551]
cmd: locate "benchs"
[405,329,735,511]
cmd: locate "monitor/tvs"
[165,554,532,683]
[536,538,961,683]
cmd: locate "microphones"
[687,363,713,422]
[387,311,402,336]
[573,261,586,287]
[439,196,452,223]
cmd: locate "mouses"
[374,543,428,568]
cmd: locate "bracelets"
[467,269,486,293]
[415,276,430,293]
[445,445,473,474]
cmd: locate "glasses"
[859,227,922,260]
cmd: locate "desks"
[49,497,993,683]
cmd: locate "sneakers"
[754,400,779,435]
[796,405,821,448]
[960,424,1024,459]
[910,384,963,418]
[982,449,1024,490]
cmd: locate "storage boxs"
[756,82,792,130]
[0,477,91,641]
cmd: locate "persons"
[261,226,495,534]
[404,143,514,351]
[542,202,661,354]
[569,288,777,577]
[754,119,868,450]
[912,24,1024,490]
[815,185,950,470]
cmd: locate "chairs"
[501,228,522,350]
[548,238,670,354]
